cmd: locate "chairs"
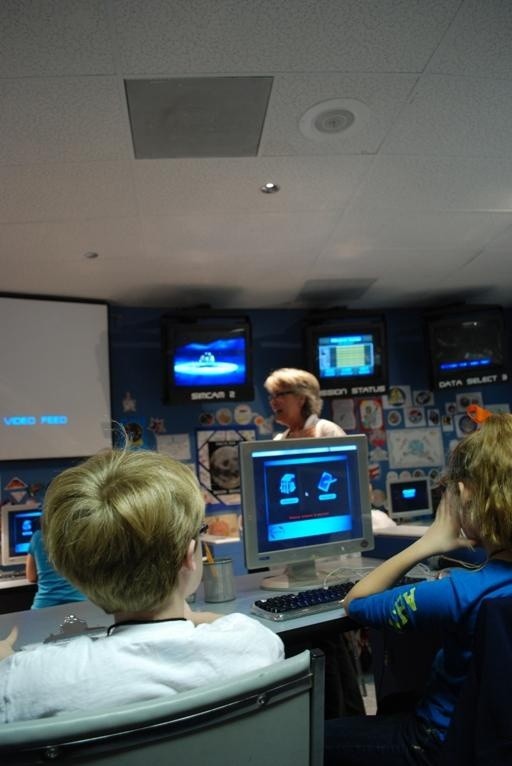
[344,594,512,766]
[0,649,316,765]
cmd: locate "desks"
[1,557,425,651]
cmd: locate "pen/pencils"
[203,540,219,577]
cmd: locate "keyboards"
[248,575,427,621]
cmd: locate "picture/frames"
[386,425,448,469]
[195,429,257,504]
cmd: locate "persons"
[1,418,286,721]
[25,531,86,610]
[263,368,346,442]
[343,414,512,762]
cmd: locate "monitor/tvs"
[294,308,391,400]
[385,476,433,518]
[238,434,375,592]
[421,304,511,393]
[0,504,43,579]
[162,304,256,407]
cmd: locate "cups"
[202,557,237,604]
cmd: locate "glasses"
[265,390,294,401]
[189,521,210,539]
